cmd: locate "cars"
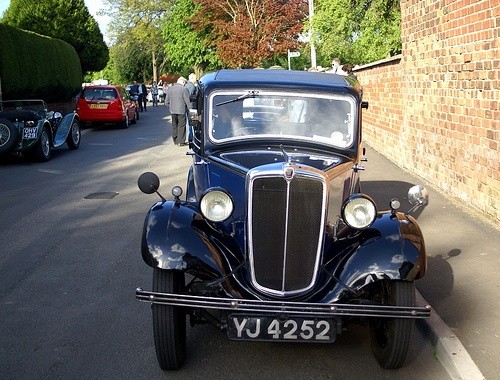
[134,70,431,370]
[0,100,80,161]
[76,86,138,128]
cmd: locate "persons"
[130,73,204,147]
[330,57,358,82]
